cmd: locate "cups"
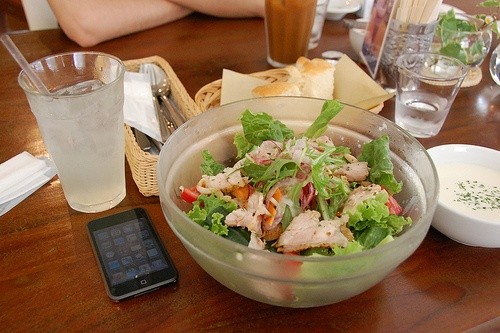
[264,0,318,69]
[349,28,367,56]
[394,52,468,139]
[17,51,126,214]
[307,0,329,50]
[381,13,440,88]
[436,13,493,76]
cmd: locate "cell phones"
[86,206,178,302]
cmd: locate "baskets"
[117,56,206,198]
[195,62,383,136]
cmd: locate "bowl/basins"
[424,143,500,249]
[157,95,440,308]
[327,0,361,22]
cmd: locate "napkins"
[0,150,57,217]
[123,72,164,144]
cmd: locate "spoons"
[146,63,184,129]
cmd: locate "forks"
[138,63,169,144]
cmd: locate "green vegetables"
[435,0,500,66]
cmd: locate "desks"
[0,1,500,333]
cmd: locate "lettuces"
[184,99,414,303]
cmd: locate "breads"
[250,57,334,102]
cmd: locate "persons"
[47,0,265,47]
